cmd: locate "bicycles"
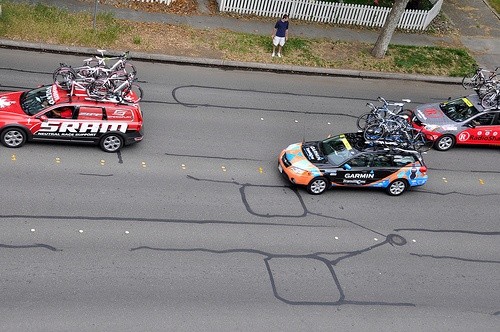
[461,62,500,111]
[53,49,144,106]
[356,96,435,156]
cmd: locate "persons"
[271,14,289,58]
[51,106,73,119]
[472,114,490,126]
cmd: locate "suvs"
[0,84,145,153]
[407,94,500,151]
[278,130,428,196]
